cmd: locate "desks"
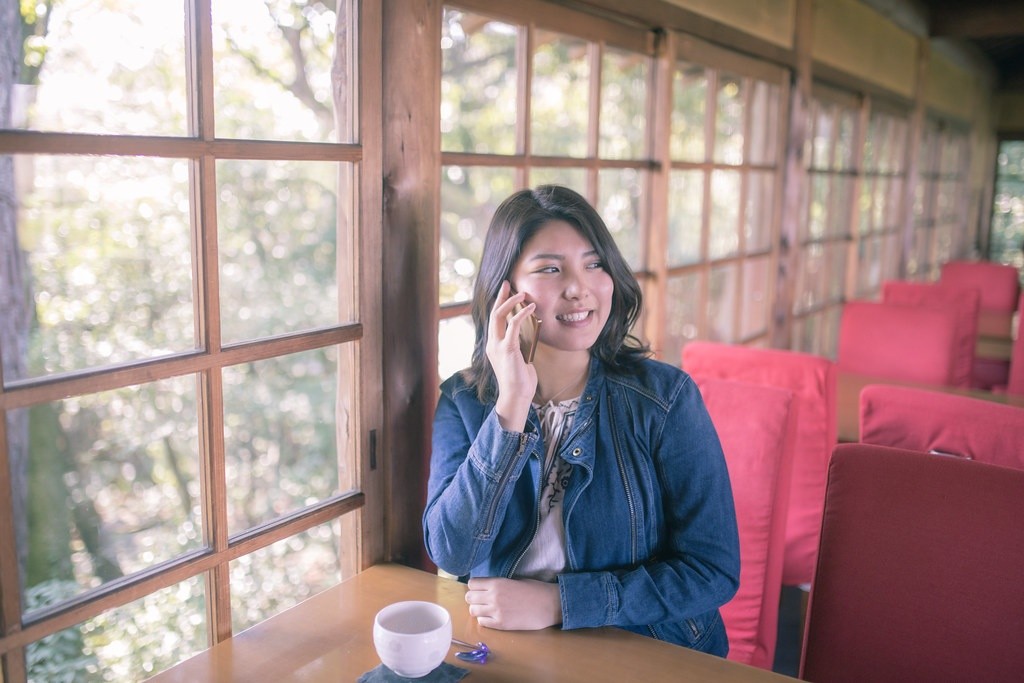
[977,310,1019,359]
[143,561,800,683]
[834,371,1024,443]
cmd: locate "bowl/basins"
[372,601,452,678]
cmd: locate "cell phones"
[490,280,543,366]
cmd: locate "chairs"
[682,261,1024,683]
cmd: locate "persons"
[424,183,740,659]
